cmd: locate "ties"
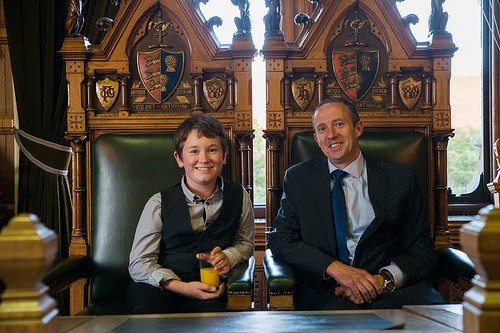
[329,169,350,267]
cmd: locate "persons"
[128,115,255,314]
[269,98,445,310]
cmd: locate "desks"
[65,303,463,333]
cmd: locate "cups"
[199,252,219,291]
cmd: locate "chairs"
[261,0,476,311]
[42,0,257,316]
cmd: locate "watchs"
[380,272,395,294]
